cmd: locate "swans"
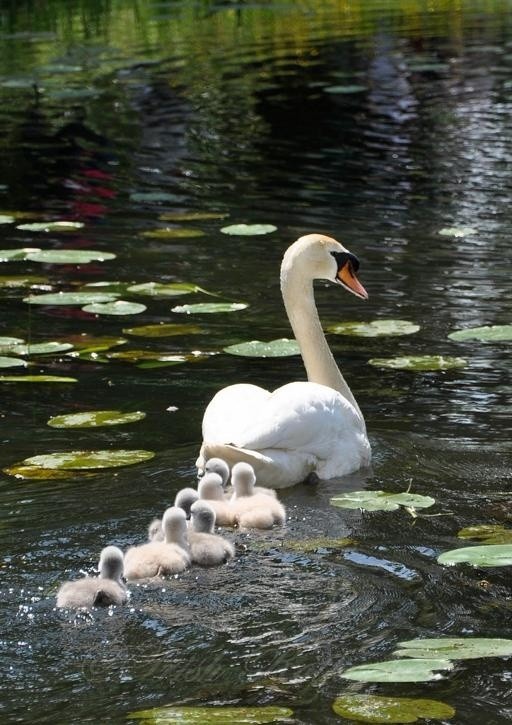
[55,457,286,611]
[192,232,374,491]
